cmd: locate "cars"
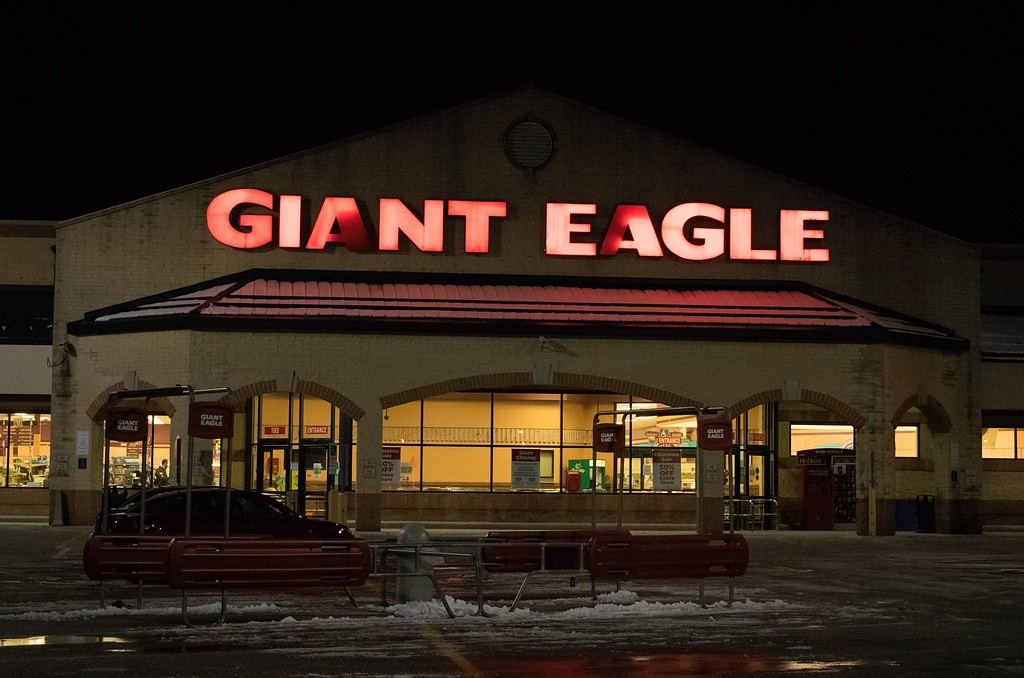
[93,485,354,542]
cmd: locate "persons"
[155,459,168,485]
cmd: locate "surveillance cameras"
[540,336,546,349]
[58,340,70,348]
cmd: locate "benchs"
[80,534,374,628]
[475,526,750,615]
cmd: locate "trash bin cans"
[397,522,433,603]
[102,484,128,510]
[916,494,936,533]
